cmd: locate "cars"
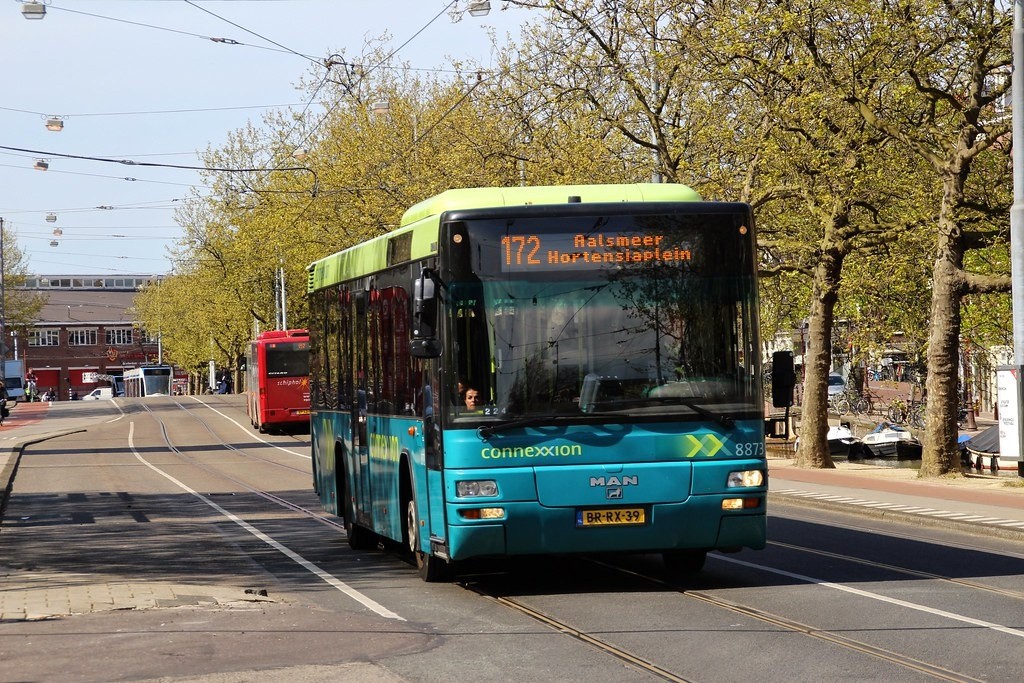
[827,371,848,407]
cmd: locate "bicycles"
[47,396,56,401]
[27,378,37,402]
[0,399,7,426]
[836,359,970,432]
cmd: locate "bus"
[237,328,311,433]
[308,183,770,583]
[123,367,173,398]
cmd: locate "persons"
[455,385,488,416]
[896,364,904,382]
[214,380,227,395]
[23,367,38,402]
[33,388,42,402]
[204,386,214,395]
[40,392,50,403]
[177,390,183,396]
[71,392,79,400]
[46,387,55,401]
[0,381,9,420]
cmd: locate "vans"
[81,388,114,401]
